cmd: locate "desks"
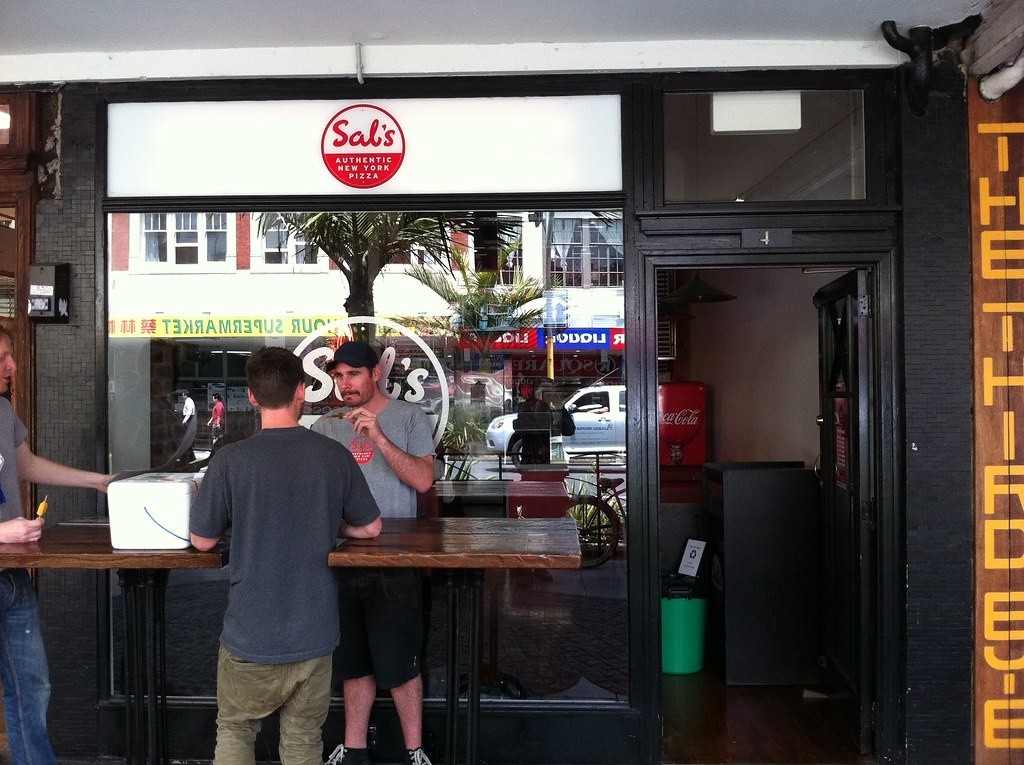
[327,517,581,765]
[0,521,232,765]
[435,481,571,699]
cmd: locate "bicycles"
[563,478,629,570]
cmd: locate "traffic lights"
[528,210,544,228]
[556,304,571,326]
[517,378,534,400]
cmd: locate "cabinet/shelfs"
[701,459,833,688]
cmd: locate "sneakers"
[405,746,433,765]
[324,744,370,765]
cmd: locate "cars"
[484,384,626,460]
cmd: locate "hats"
[326,341,378,372]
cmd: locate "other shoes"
[512,568,553,583]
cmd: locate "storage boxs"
[107,472,206,550]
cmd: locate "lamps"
[657,271,736,321]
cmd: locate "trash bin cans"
[661,570,708,675]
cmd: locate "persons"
[310,342,437,765]
[513,379,576,581]
[588,395,608,414]
[208,394,224,444]
[190,346,382,765]
[182,390,196,448]
[0,325,120,765]
